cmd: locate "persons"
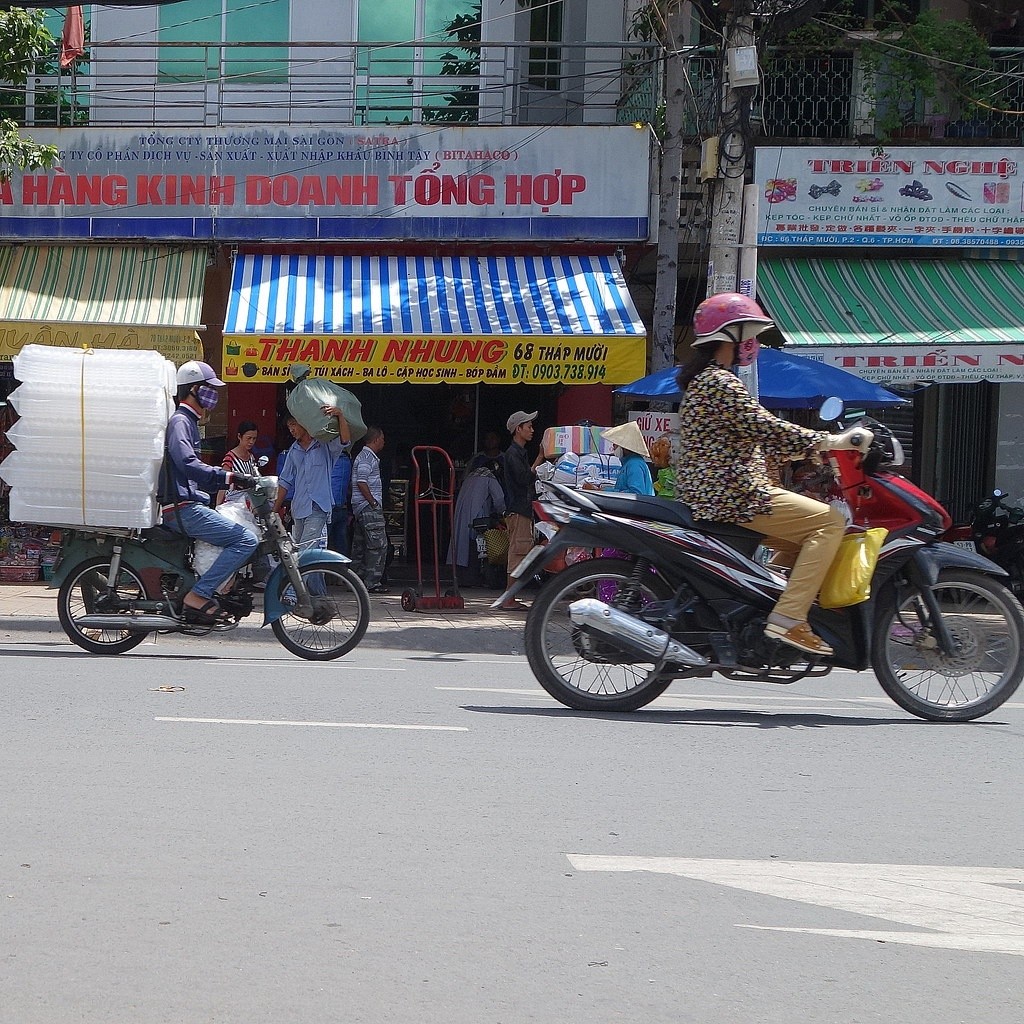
[675,292,874,655]
[447,424,505,589]
[499,410,544,608]
[265,407,352,613]
[215,423,262,513]
[352,425,393,594]
[583,420,657,608]
[157,361,254,620]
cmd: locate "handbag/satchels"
[818,523,889,609]
[483,511,509,566]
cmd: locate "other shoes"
[455,577,476,586]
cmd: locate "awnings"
[222,249,646,386]
[0,240,211,368]
[754,256,1023,384]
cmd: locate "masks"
[736,339,759,366]
[196,385,219,411]
[614,446,624,458]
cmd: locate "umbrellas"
[611,342,910,408]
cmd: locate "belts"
[333,504,347,509]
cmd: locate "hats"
[177,360,226,387]
[690,322,775,350]
[507,410,538,435]
[599,421,651,458]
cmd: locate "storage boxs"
[383,479,409,561]
[0,562,53,581]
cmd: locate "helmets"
[693,293,773,337]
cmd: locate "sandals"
[368,584,392,593]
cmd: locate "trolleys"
[401,445,477,616]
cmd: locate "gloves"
[827,428,874,453]
[228,470,257,491]
[788,447,821,461]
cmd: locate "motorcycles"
[930,489,1024,611]
[45,454,371,661]
[488,397,1023,723]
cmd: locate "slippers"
[764,620,833,655]
[183,600,233,618]
[497,604,528,611]
